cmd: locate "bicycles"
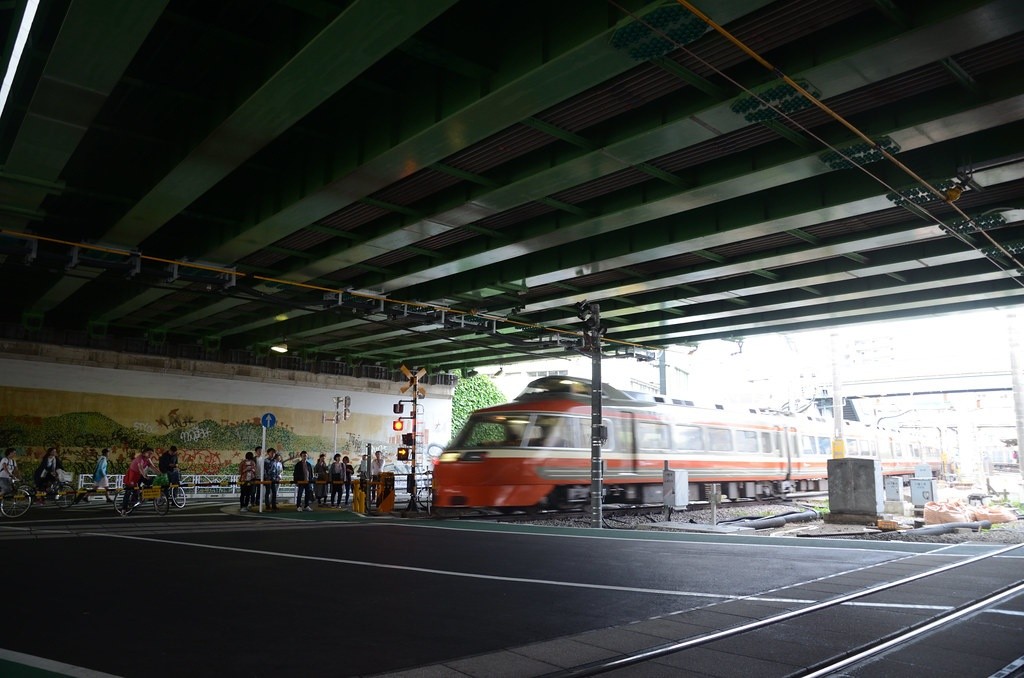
[114,474,169,516]
[18,472,76,509]
[418,471,433,508]
[156,467,188,509]
[0,478,32,519]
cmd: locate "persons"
[122,447,154,516]
[0,448,19,501]
[292,450,385,513]
[159,446,181,506]
[83,449,115,503]
[239,446,283,513]
[34,448,63,505]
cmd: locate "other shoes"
[82,496,89,504]
[136,506,145,511]
[106,498,114,503]
[322,503,328,506]
[297,506,302,512]
[345,501,349,506]
[372,499,375,504]
[331,504,336,509]
[38,498,47,507]
[121,502,132,510]
[171,501,181,506]
[251,503,254,507]
[305,506,313,511]
[240,507,248,512]
[336,504,341,508]
[255,501,260,506]
[273,506,280,511]
[318,504,323,507]
[266,506,271,510]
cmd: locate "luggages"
[71,482,93,505]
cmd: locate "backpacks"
[33,457,54,484]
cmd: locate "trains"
[432,376,970,515]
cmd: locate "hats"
[101,448,110,453]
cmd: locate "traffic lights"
[393,421,403,430]
[402,434,411,445]
[398,448,408,460]
